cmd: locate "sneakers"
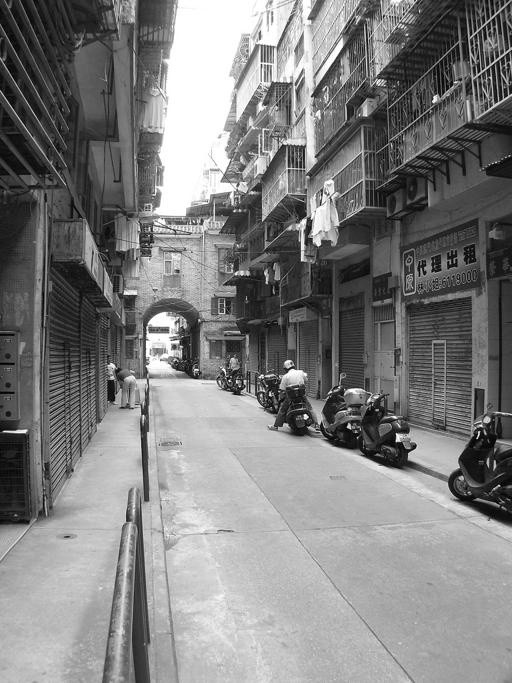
[107,400,118,405]
[315,424,321,431]
[268,425,278,430]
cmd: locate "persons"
[113,367,138,409]
[227,353,242,372]
[105,354,120,406]
[267,358,321,430]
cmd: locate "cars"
[159,353,180,368]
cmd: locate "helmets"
[282,359,295,370]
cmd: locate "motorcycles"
[317,371,367,446]
[282,382,313,436]
[252,367,282,412]
[445,402,511,520]
[215,354,246,394]
[357,388,417,469]
[174,359,200,378]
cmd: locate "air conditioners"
[355,97,377,120]
